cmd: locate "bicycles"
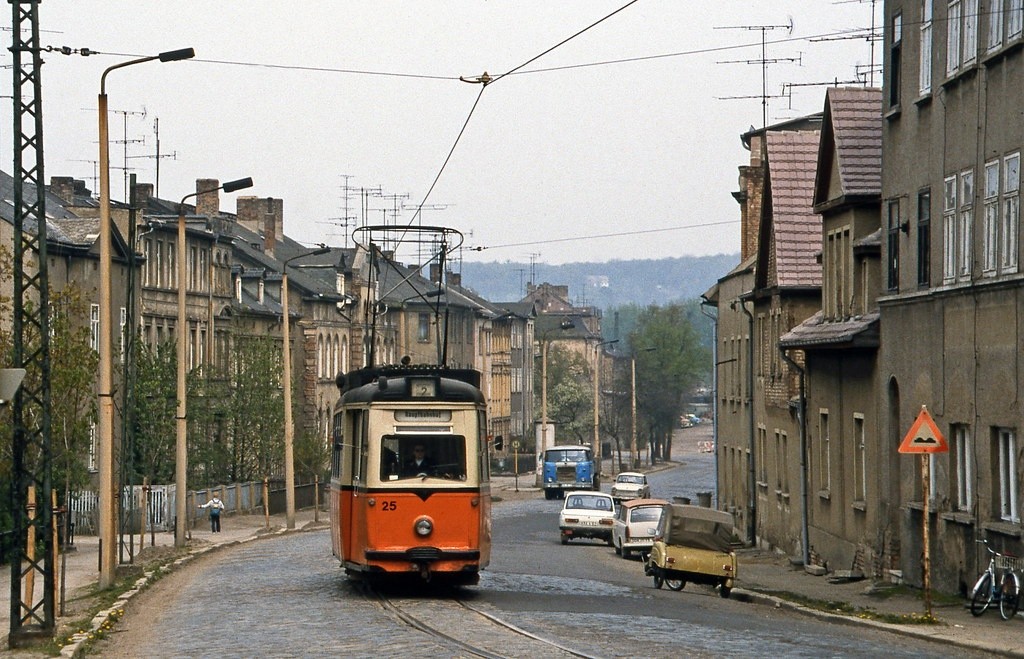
[970,540,1024,621]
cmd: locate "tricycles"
[645,504,737,598]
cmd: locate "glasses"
[415,449,424,452]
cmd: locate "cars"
[698,435,714,453]
[612,499,673,558]
[680,414,704,429]
[558,491,616,546]
[611,472,650,505]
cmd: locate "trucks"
[539,445,602,500]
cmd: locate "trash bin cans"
[671,495,692,504]
[697,491,712,508]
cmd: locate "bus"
[330,225,503,596]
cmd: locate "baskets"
[995,555,1024,571]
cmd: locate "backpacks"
[209,499,222,517]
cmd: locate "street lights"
[400,288,445,364]
[282,247,331,529]
[480,312,514,404]
[174,177,254,547]
[542,324,575,456]
[594,340,619,457]
[630,348,656,470]
[98,47,195,590]
